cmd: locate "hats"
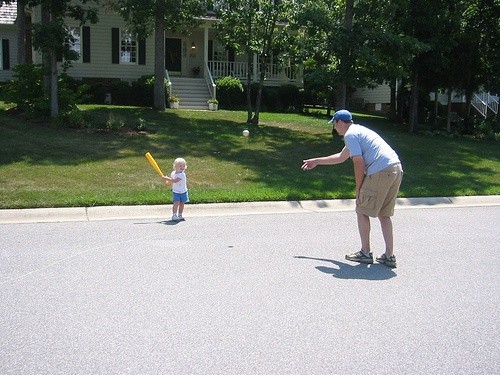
[328,109,352,124]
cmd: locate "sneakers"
[345,248,373,264]
[172,215,179,221]
[178,215,184,220]
[376,254,397,268]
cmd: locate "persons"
[302,110,403,267]
[162,158,190,222]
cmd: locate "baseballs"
[243,129,249,136]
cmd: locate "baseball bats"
[145,152,169,184]
